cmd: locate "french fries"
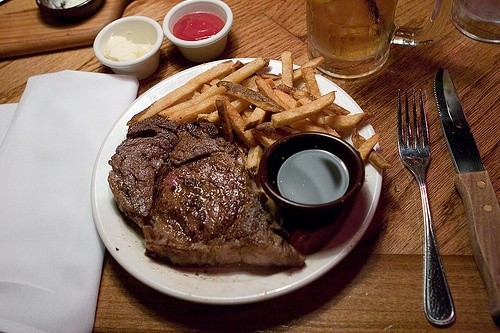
[126,51,391,217]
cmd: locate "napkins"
[0,67,140,333]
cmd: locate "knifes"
[434,68,500,324]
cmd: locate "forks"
[396,88,456,325]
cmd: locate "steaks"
[108,117,307,268]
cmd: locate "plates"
[90,58,384,304]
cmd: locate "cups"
[162,1,233,63]
[302,0,453,78]
[453,0,500,44]
[257,132,365,234]
[93,16,164,80]
